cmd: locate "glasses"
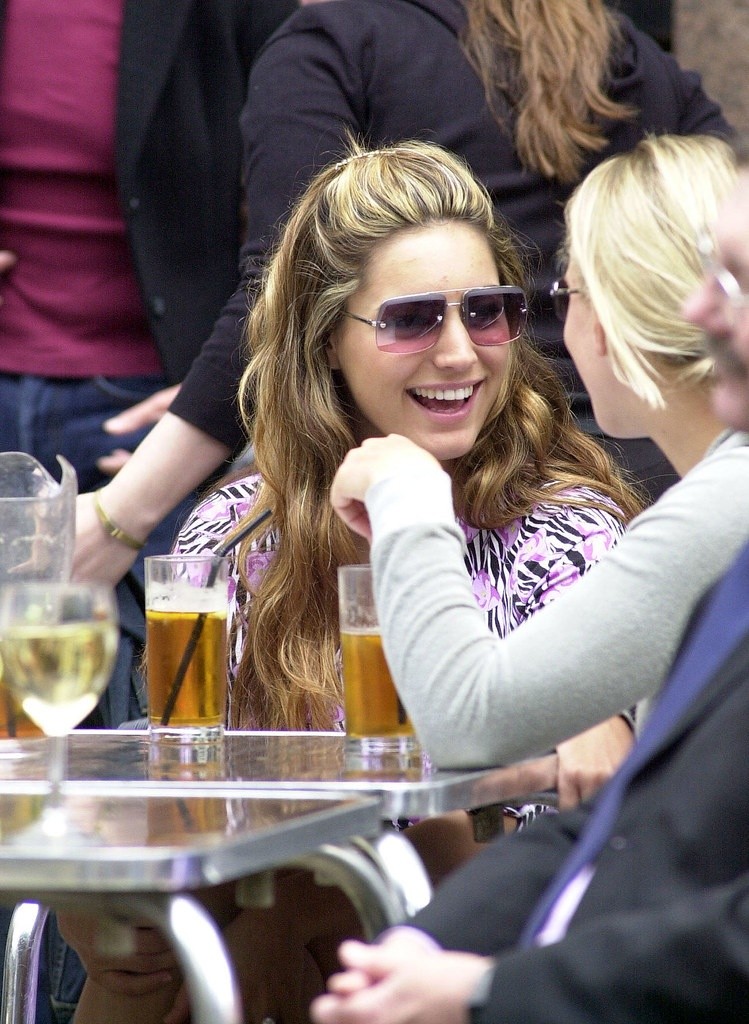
[341,285,529,355]
[549,276,588,322]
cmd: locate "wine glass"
[0,579,120,847]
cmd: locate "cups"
[143,555,232,745]
[338,564,424,755]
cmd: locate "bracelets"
[93,488,152,552]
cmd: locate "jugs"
[0,452,78,754]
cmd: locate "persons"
[331,135,749,768]
[52,141,651,1024]
[305,136,749,1024]
[68,0,748,593]
[0,0,305,728]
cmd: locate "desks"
[0,730,561,922]
[0,782,405,1024]
